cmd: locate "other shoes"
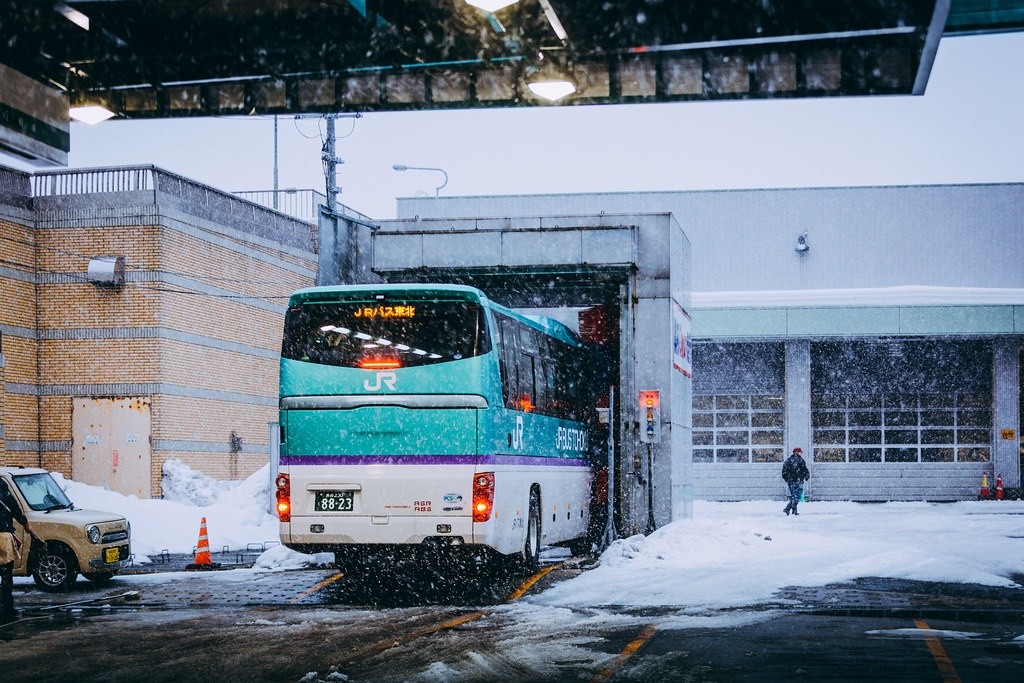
[1,617,20,624]
[10,610,20,615]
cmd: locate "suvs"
[0,465,131,592]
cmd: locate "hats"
[793,448,803,453]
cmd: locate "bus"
[276,283,612,574]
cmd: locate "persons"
[781,448,810,516]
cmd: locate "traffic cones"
[994,474,1006,500]
[186,518,221,569]
[977,473,990,501]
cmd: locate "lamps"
[526,57,578,102]
[794,233,809,252]
[68,94,115,126]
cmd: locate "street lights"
[393,165,448,198]
